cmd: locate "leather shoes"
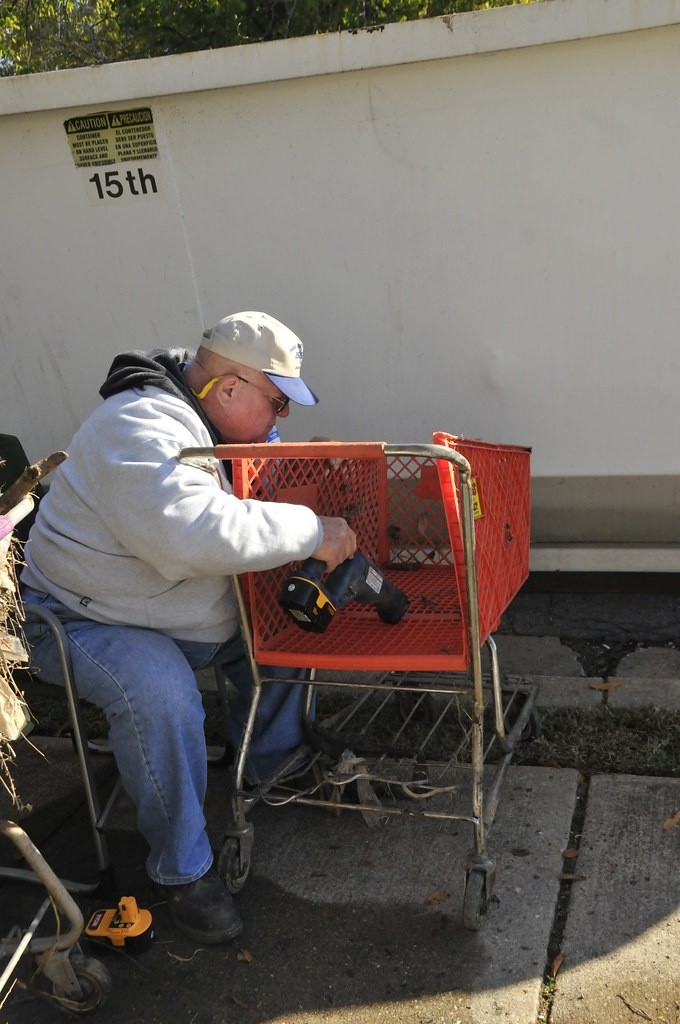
[243,761,336,802]
[150,858,244,945]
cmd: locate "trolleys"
[180,431,542,932]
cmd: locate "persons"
[17,310,359,941]
[0,433,46,580]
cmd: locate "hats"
[200,310,319,406]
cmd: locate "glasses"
[236,375,290,413]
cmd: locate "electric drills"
[276,549,411,634]
